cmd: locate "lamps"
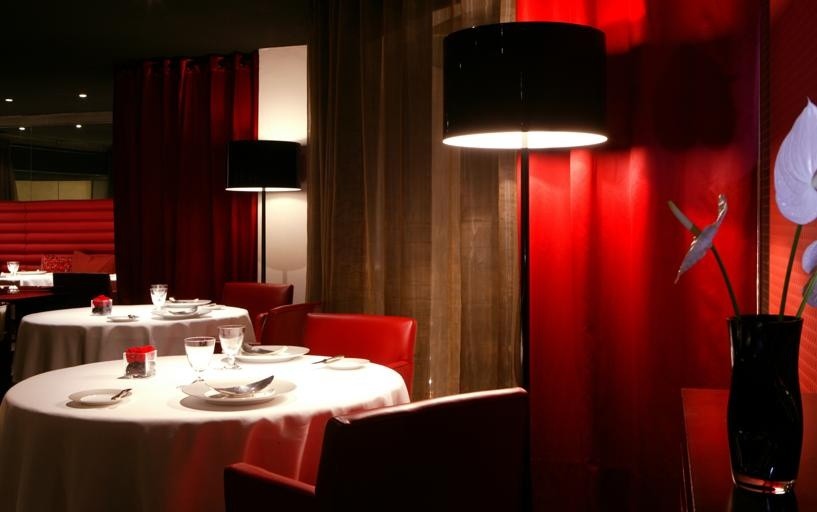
[226,139,302,283]
[441,22,610,388]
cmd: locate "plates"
[16,269,48,276]
[181,375,300,407]
[325,357,370,371]
[107,295,225,323]
[232,342,311,364]
[67,388,134,407]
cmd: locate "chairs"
[220,384,526,512]
[254,301,322,346]
[222,281,293,329]
[303,312,420,399]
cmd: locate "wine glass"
[150,282,169,294]
[183,335,216,385]
[216,325,245,375]
[6,260,20,280]
[149,288,169,320]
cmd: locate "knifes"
[311,354,345,365]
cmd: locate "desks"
[680,386,817,512]
[1,281,53,310]
[8,301,257,385]
[2,343,412,510]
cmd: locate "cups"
[89,299,113,315]
[7,285,21,294]
[121,349,157,378]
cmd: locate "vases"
[726,318,803,495]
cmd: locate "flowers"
[670,93,817,315]
